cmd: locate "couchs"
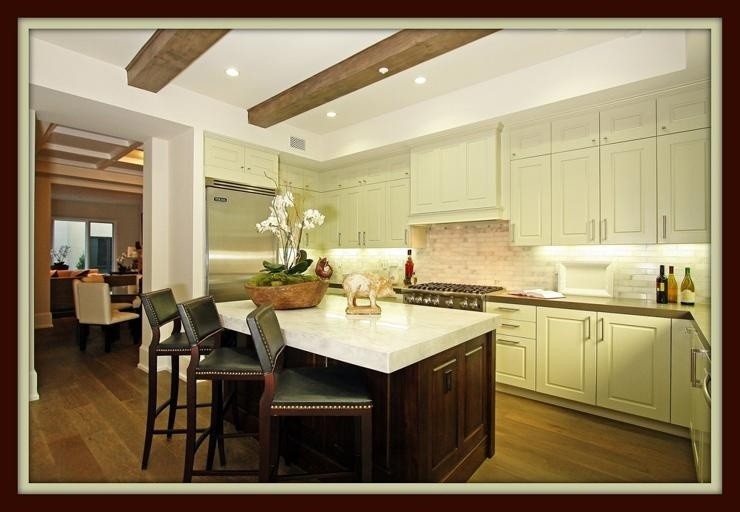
[50,268,100,311]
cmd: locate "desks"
[215,294,495,483]
[109,284,141,340]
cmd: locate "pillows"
[75,270,89,277]
[57,270,71,277]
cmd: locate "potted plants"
[49,246,72,270]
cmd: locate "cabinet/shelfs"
[552,91,655,245]
[319,184,342,249]
[485,302,536,393]
[303,185,319,249]
[688,320,711,483]
[204,133,279,188]
[386,169,427,248]
[509,118,552,245]
[656,80,711,244]
[537,306,690,425]
[342,176,386,248]
[406,130,509,223]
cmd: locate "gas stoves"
[402,282,505,311]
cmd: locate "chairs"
[139,288,242,470]
[245,303,374,482]
[176,296,264,483]
[77,283,141,353]
[72,278,133,341]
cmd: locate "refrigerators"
[205,176,280,300]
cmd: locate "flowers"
[250,170,326,286]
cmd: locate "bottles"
[405,249,414,286]
[410,273,417,286]
[681,267,695,306]
[655,265,668,305]
[667,266,678,304]
